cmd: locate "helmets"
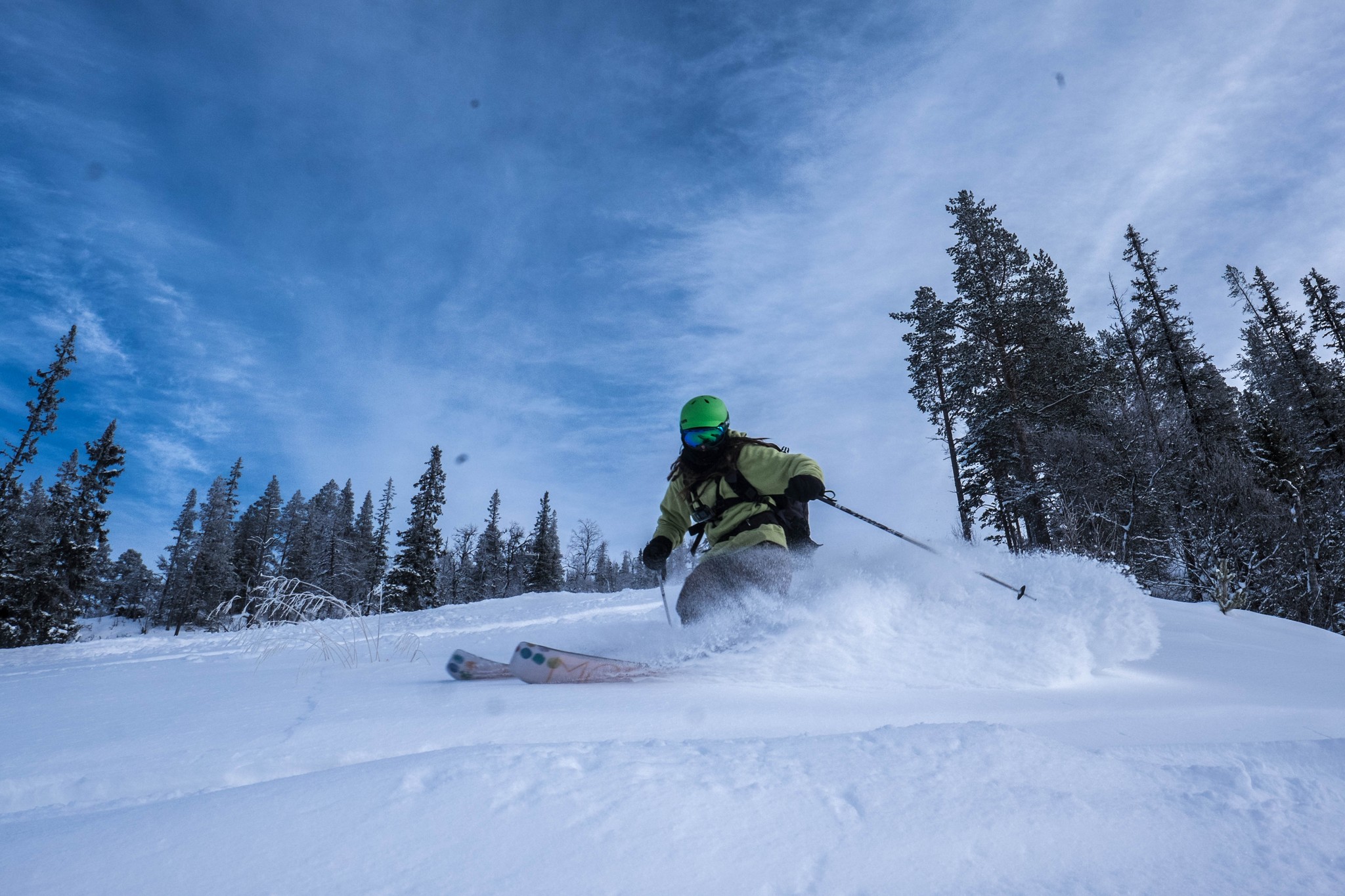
[680,395,730,452]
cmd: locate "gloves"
[784,475,825,504]
[643,535,673,570]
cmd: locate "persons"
[642,395,825,627]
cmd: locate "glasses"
[683,426,723,450]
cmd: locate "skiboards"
[445,642,654,684]
